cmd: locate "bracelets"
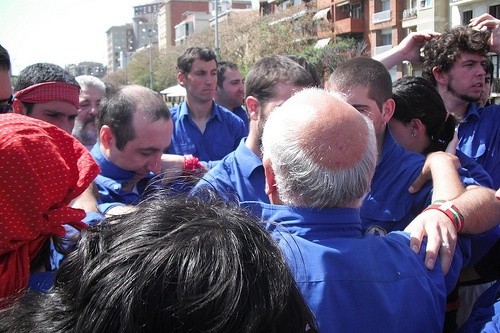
[421,199,465,232]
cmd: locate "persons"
[0,11,500,333]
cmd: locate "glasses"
[0,96,15,113]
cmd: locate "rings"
[439,242,451,248]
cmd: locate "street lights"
[146,28,157,90]
